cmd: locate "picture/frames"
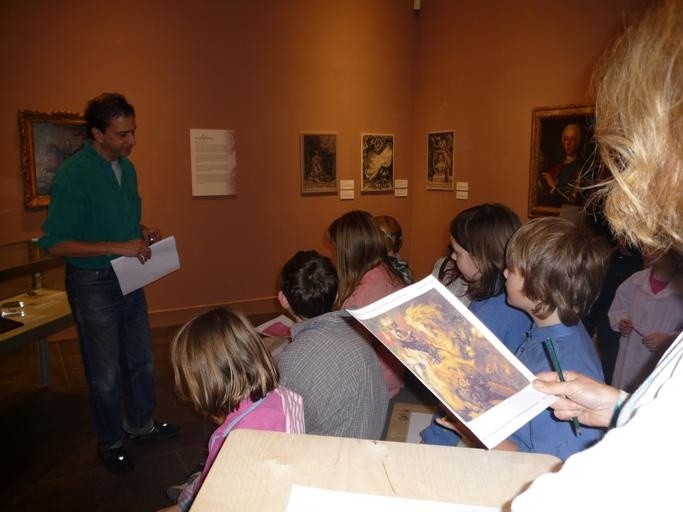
[16,108,90,212]
[423,130,455,191]
[358,131,395,194]
[298,129,338,196]
[524,104,610,224]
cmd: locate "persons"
[508,3,681,510]
[326,209,407,415]
[166,304,307,510]
[418,201,537,448]
[36,91,181,473]
[606,240,682,393]
[434,215,609,463]
[365,214,412,292]
[395,328,441,364]
[539,123,591,210]
[428,237,478,313]
[273,248,389,441]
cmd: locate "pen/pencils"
[624,321,646,339]
[545,336,583,437]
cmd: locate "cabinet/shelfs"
[0,235,80,389]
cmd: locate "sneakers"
[98,449,133,472]
[124,420,179,442]
